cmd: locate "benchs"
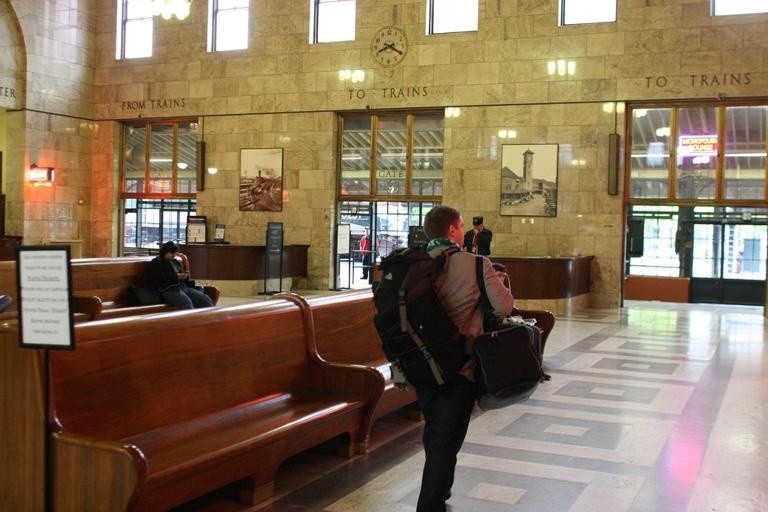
[2,256,219,325]
[2,287,424,510]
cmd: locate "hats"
[472,216,483,226]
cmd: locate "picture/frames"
[238,149,284,212]
[185,216,284,253]
[498,143,560,217]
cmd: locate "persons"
[359,227,380,279]
[492,262,551,383]
[151,241,214,312]
[463,217,492,255]
[416,206,513,511]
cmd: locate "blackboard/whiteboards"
[267,222,284,255]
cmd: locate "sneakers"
[541,368,550,380]
[361,276,367,279]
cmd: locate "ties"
[474,230,480,244]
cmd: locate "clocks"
[367,24,410,72]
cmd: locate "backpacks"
[371,242,477,393]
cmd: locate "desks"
[491,255,595,317]
[159,243,311,298]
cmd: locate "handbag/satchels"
[474,255,550,412]
[127,282,162,306]
